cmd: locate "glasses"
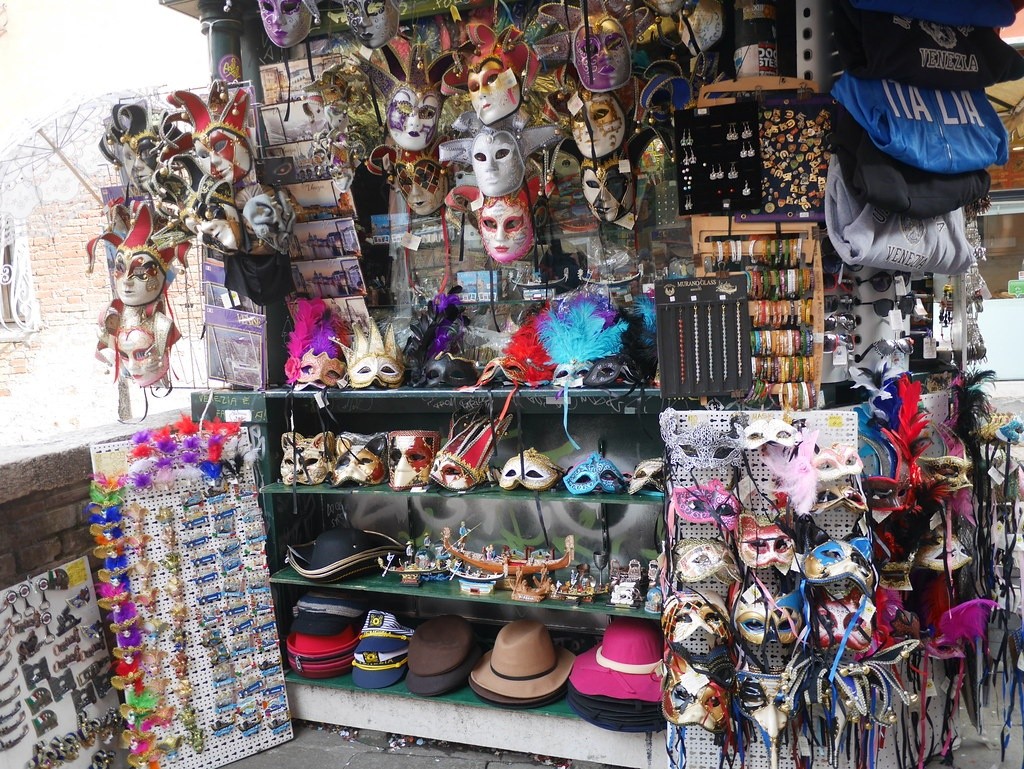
[822,239,917,364]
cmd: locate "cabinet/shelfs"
[147,0,1024,769]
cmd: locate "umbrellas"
[0,87,161,220]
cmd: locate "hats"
[284,523,671,733]
